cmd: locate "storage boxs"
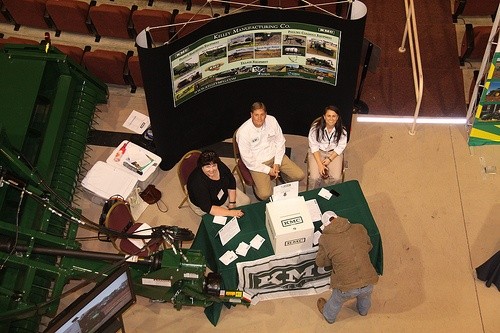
[265,194,315,256]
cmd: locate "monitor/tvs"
[44,265,136,333]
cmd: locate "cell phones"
[330,189,340,197]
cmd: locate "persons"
[307,106,347,189]
[316,210,378,325]
[186,150,250,219]
[236,102,305,200]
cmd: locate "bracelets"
[229,201,236,203]
[328,157,332,162]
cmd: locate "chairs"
[304,146,348,192]
[0,0,354,94]
[103,200,167,264]
[466,70,489,112]
[230,127,279,194]
[176,150,203,209]
[451,0,500,23]
[458,22,500,67]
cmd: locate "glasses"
[199,152,217,158]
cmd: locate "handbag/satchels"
[138,184,162,205]
[99,194,131,227]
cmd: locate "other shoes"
[279,176,286,183]
[317,298,335,324]
[357,305,367,315]
[252,185,262,201]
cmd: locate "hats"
[321,211,337,226]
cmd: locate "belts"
[320,149,335,152]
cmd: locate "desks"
[79,160,149,220]
[187,179,384,327]
[105,139,163,191]
